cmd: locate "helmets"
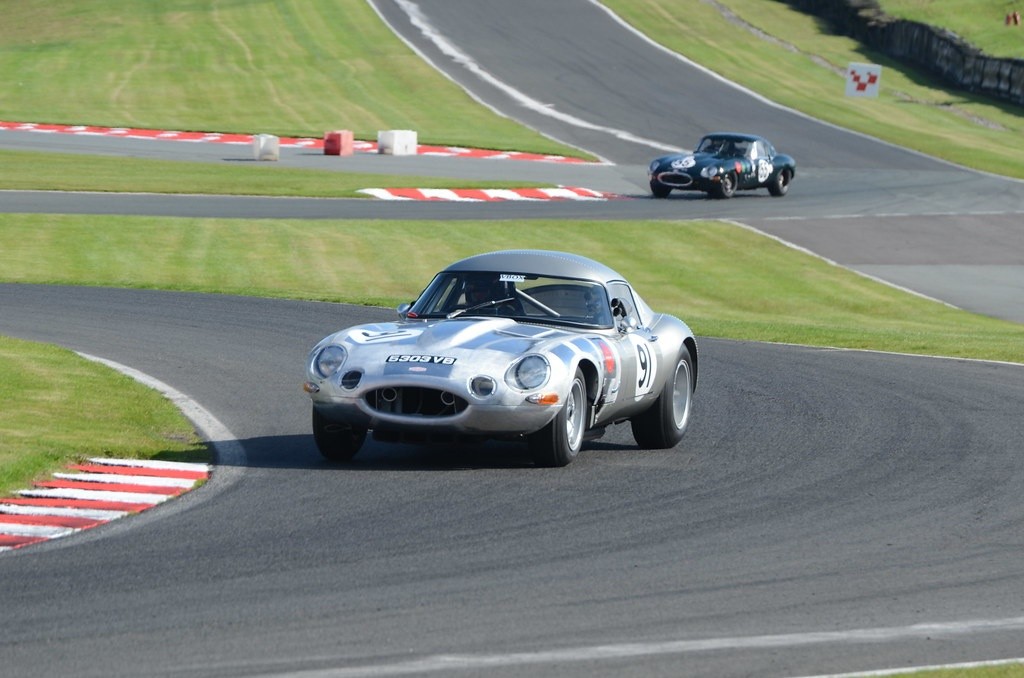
[465,274,492,305]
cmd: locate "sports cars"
[648,132,797,200]
[303,249,699,466]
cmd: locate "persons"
[465,279,505,308]
[713,140,721,151]
